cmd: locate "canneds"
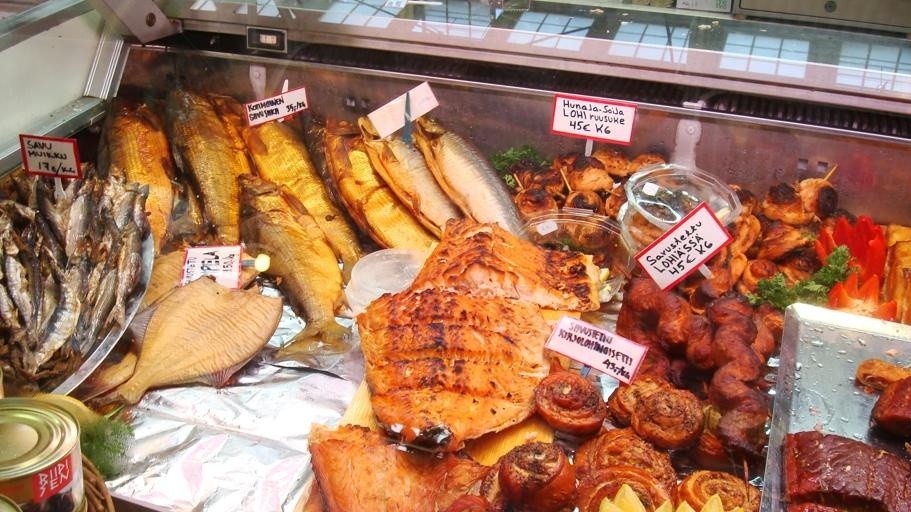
[0,495,21,512]
[0,398,88,512]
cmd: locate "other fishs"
[2,83,535,410]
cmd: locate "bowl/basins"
[511,207,634,304]
